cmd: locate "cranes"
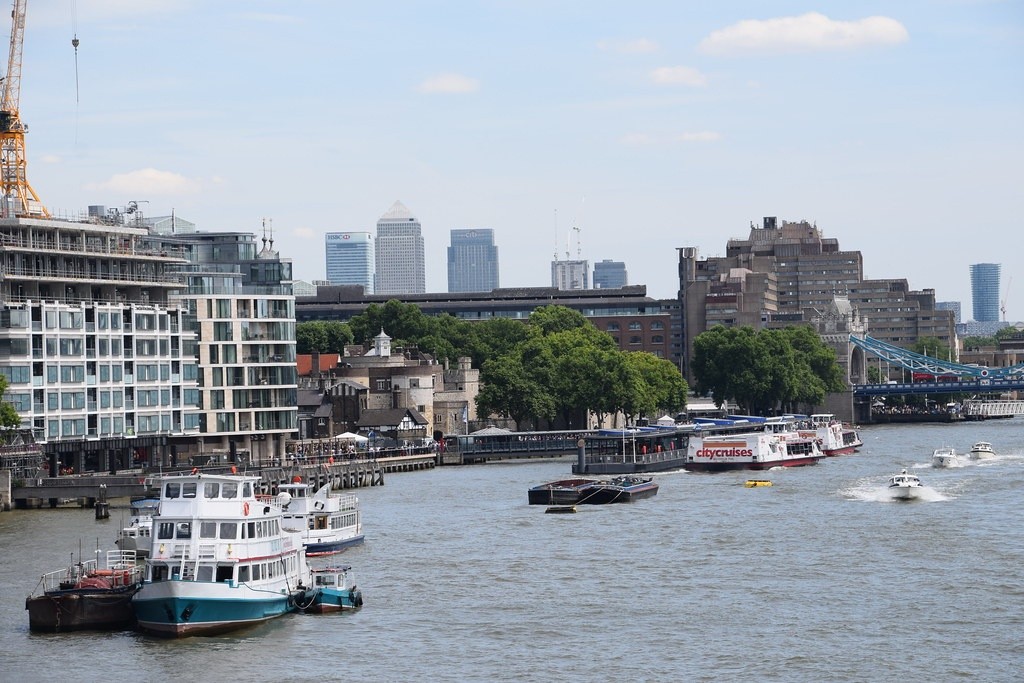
[2,0,81,217]
[1001,275,1013,321]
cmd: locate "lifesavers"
[244,502,250,515]
[802,434,807,439]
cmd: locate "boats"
[888,468,923,499]
[586,476,659,503]
[528,479,600,507]
[132,472,312,638]
[971,441,997,459]
[307,564,363,614]
[116,498,162,559]
[685,431,827,473]
[810,413,852,428]
[933,442,959,467]
[277,476,365,555]
[784,419,863,458]
[25,538,147,636]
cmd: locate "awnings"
[657,415,675,426]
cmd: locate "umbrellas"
[332,432,369,442]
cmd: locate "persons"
[477,433,592,443]
[874,400,963,414]
[771,418,842,431]
[268,441,451,463]
